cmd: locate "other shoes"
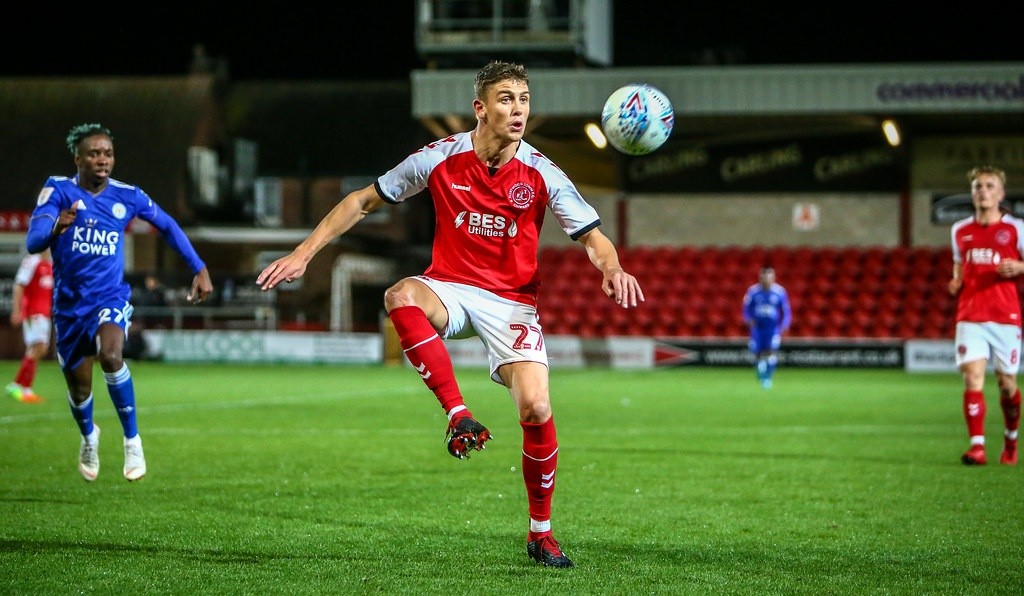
[6,382,46,404]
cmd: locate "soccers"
[602,83,675,156]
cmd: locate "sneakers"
[444,409,493,459]
[963,444,986,464]
[527,531,573,567]
[78,423,100,481]
[1000,436,1018,464]
[123,433,147,481]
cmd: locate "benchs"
[535,243,958,339]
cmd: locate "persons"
[26,122,213,482]
[4,246,54,403]
[741,264,791,389]
[948,166,1024,462]
[254,61,644,568]
